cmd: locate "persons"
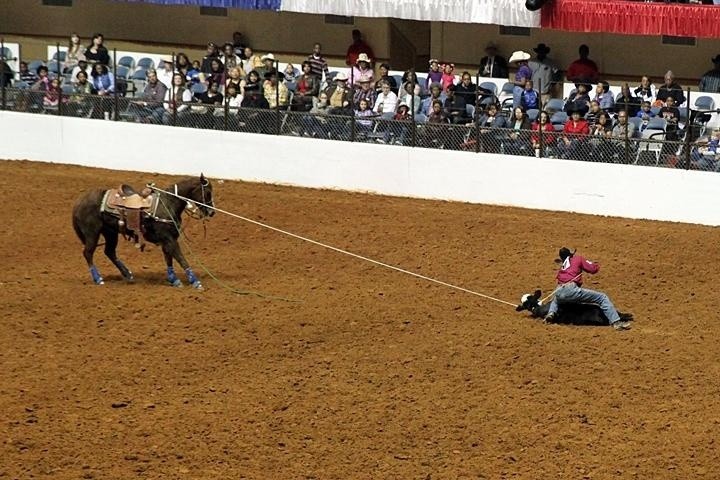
[294,28,506,155]
[502,41,720,173]
[126,31,297,135]
[0,31,120,121]
[541,246,631,330]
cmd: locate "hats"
[397,102,410,112]
[357,54,371,65]
[160,55,174,63]
[260,54,277,63]
[534,44,550,54]
[243,82,259,91]
[355,75,374,84]
[508,51,531,63]
[333,73,350,82]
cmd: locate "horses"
[71,171,216,292]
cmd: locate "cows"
[514,289,634,326]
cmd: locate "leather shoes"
[613,320,631,331]
[543,315,551,322]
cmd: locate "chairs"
[0,46,204,125]
[280,67,713,172]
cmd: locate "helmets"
[559,246,571,260]
